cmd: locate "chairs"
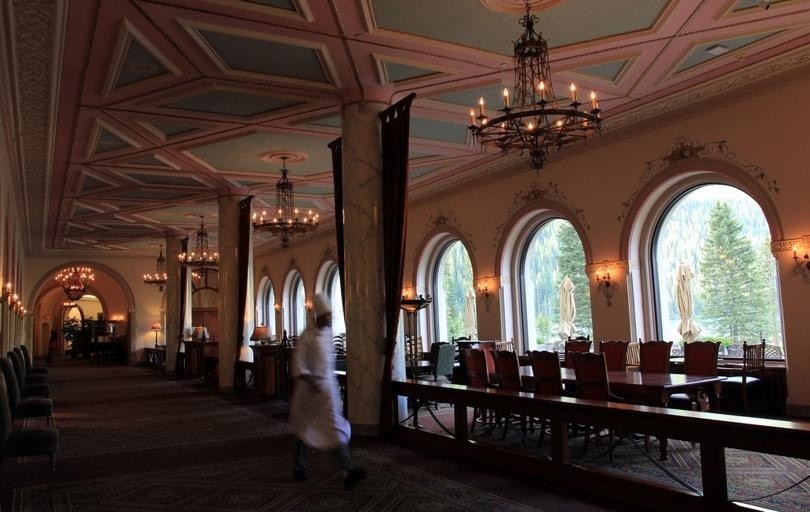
[1,338,62,511]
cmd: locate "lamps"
[191,326,210,340]
[52,266,96,302]
[0,274,29,326]
[463,3,605,170]
[152,321,162,346]
[142,242,168,291]
[249,326,272,344]
[248,154,320,248]
[400,293,434,434]
[476,281,491,298]
[595,268,613,290]
[791,242,810,275]
[177,212,221,267]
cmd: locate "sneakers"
[347,466,370,478]
[293,466,307,482]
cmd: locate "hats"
[311,293,332,318]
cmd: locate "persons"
[290,311,366,490]
